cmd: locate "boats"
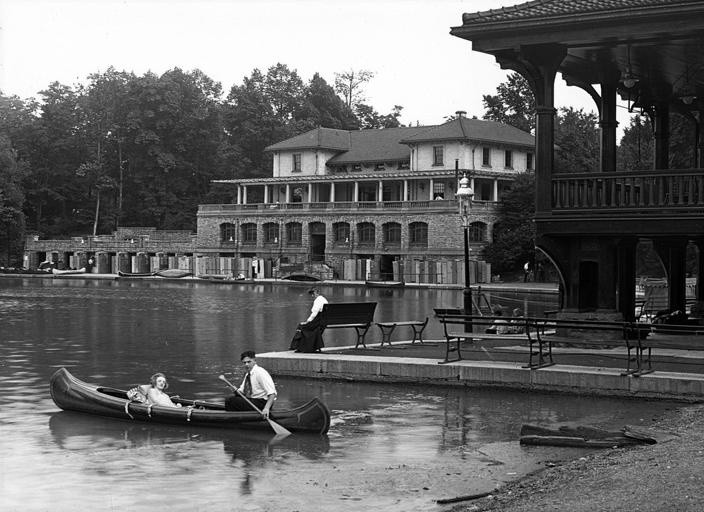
[51,266,227,280]
[432,305,510,318]
[49,365,330,434]
[364,280,405,288]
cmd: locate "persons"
[504,307,526,334]
[482,304,509,334]
[223,350,276,421]
[434,193,446,200]
[287,286,328,353]
[233,272,245,281]
[144,371,182,409]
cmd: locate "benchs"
[296,300,430,352]
[437,313,703,377]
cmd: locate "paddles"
[219,375,291,436]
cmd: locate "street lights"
[454,171,476,350]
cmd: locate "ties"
[243,372,253,396]
